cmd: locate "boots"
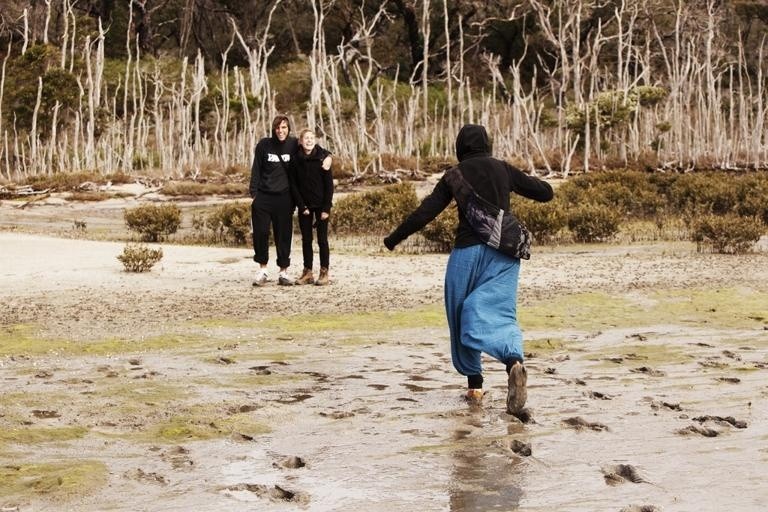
[295,267,315,285]
[314,268,330,286]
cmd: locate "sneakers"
[465,388,484,402]
[276,271,290,285]
[252,268,273,286]
[505,361,531,416]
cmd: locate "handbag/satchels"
[442,165,533,259]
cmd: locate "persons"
[249,116,333,286]
[384,123,553,415]
[295,130,334,286]
